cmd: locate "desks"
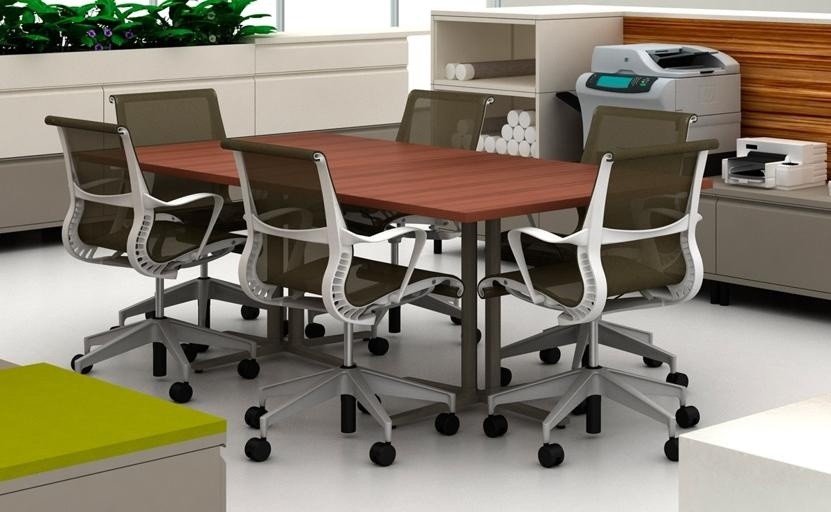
[78,132,714,431]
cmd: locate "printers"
[554,43,742,181]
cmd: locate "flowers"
[1,1,278,55]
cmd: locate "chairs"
[477,139,719,469]
[219,139,465,467]
[45,115,258,403]
[109,88,260,327]
[502,106,698,386]
[365,90,495,355]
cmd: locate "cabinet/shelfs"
[0,363,228,511]
[680,395,831,512]
[430,11,623,244]
[539,175,831,314]
